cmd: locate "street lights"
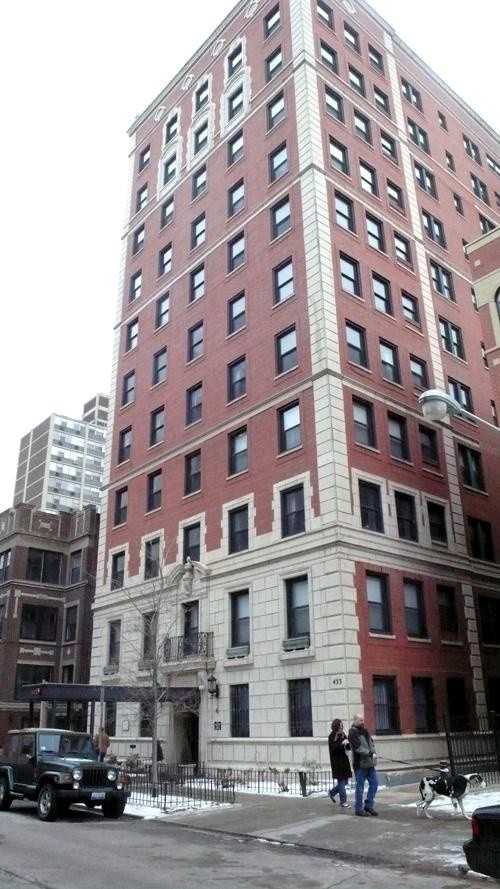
[416,387,500,433]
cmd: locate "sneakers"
[341,803,352,809]
[364,807,377,816]
[356,811,369,817]
[328,793,336,803]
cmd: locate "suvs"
[0,725,134,821]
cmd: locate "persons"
[348,713,379,816]
[94,727,110,762]
[328,719,353,809]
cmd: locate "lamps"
[207,672,218,696]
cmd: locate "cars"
[461,801,500,882]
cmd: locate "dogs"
[415,771,487,821]
